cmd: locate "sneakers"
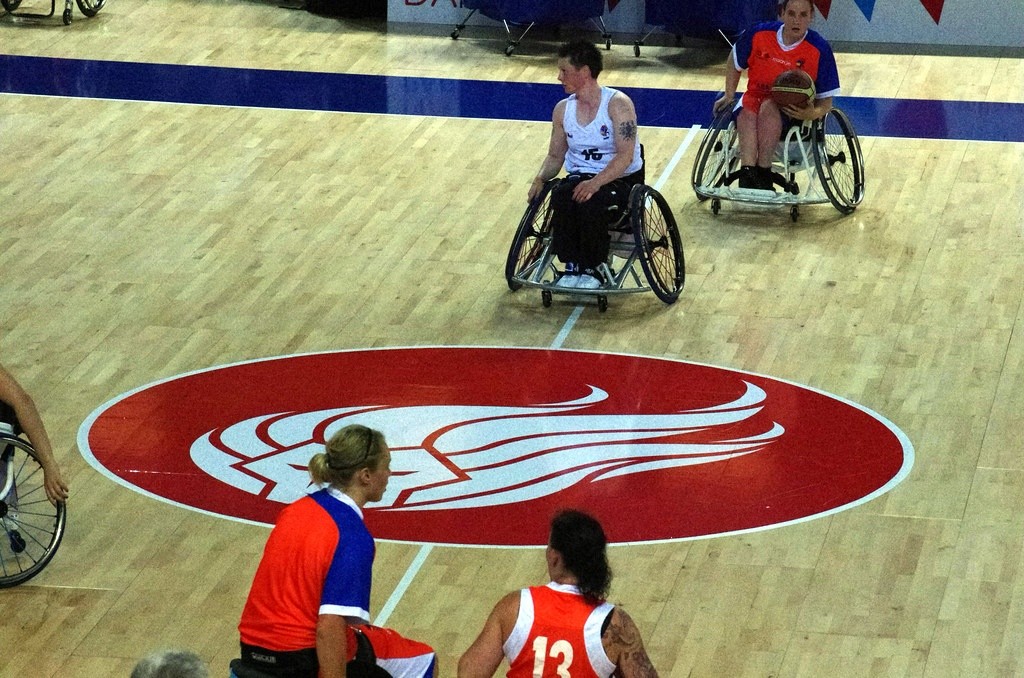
[555,264,604,290]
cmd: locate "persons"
[526,39,644,289]
[237,423,439,678]
[711,0,841,192]
[0,364,69,508]
[456,508,660,678]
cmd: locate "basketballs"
[772,71,816,107]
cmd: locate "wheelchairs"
[504,142,688,311]
[691,94,869,221]
[0,395,70,590]
[0,0,106,27]
